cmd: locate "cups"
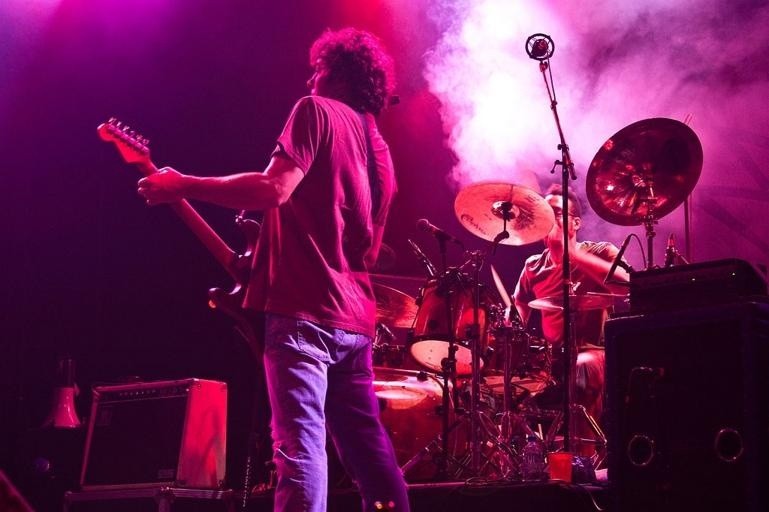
[548,452,573,484]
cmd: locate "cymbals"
[586,119,701,225]
[529,290,623,314]
[370,282,421,330]
[456,181,556,245]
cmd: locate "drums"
[485,327,553,398]
[326,365,467,486]
[408,275,501,377]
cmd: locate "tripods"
[401,231,606,487]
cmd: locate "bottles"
[522,435,545,481]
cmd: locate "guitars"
[95,118,262,358]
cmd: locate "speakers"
[78,378,228,491]
[604,300,768,512]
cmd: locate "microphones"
[525,33,556,60]
[416,218,453,241]
[605,233,631,284]
[377,322,398,342]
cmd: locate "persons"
[503,180,630,483]
[136,28,411,512]
